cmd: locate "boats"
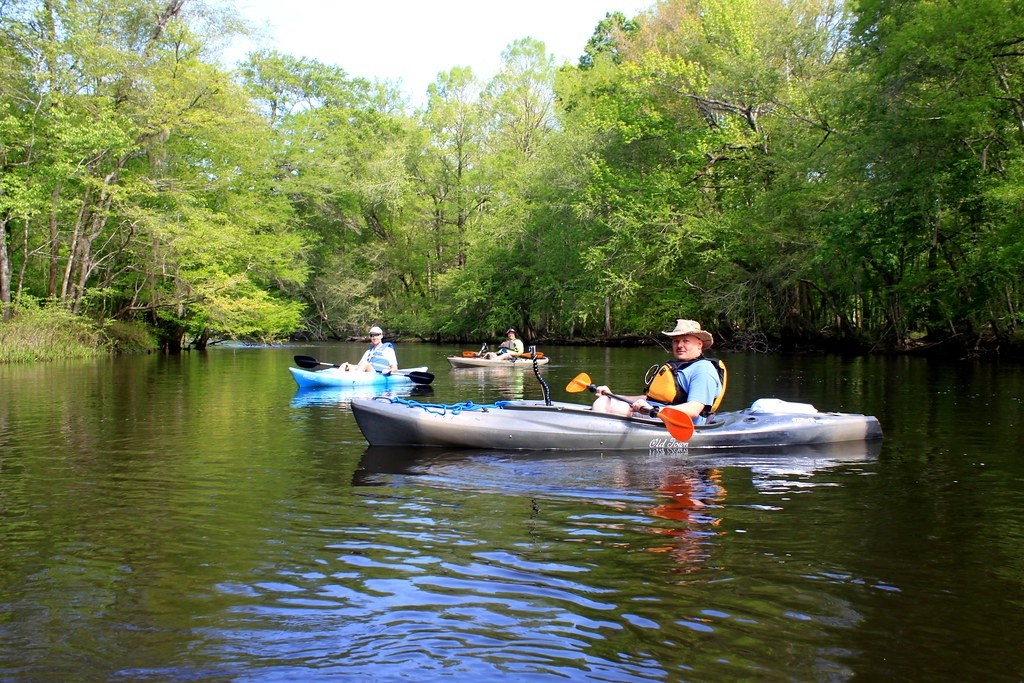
[288,386,413,408]
[350,443,874,498]
[352,397,883,452]
[446,356,549,369]
[289,367,428,388]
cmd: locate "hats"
[505,329,518,338]
[370,327,382,334]
[662,319,714,351]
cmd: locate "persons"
[592,319,722,423]
[492,329,524,360]
[338,327,399,373]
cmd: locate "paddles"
[566,371,693,442]
[462,350,544,358]
[294,354,434,384]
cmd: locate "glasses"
[370,333,381,337]
